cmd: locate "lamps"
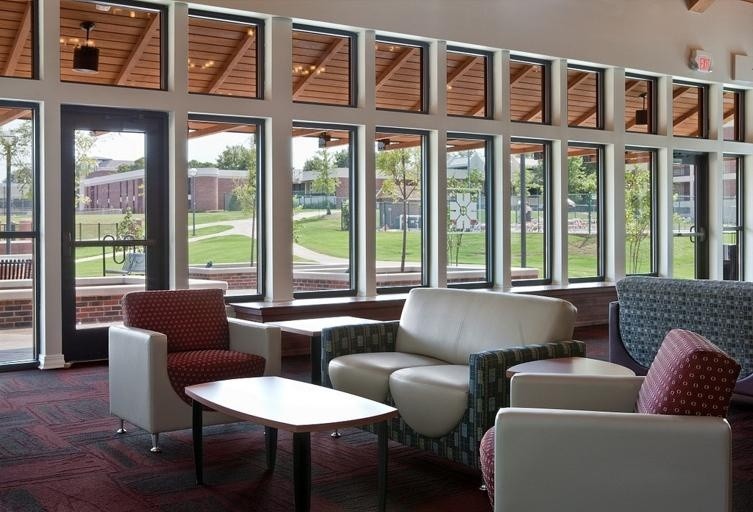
[634,92,647,125]
[72,22,100,74]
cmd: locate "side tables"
[265,316,381,385]
[505,356,636,380]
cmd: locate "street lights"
[190,168,197,236]
[2,133,19,256]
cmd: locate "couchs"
[609,276,753,397]
[321,287,587,471]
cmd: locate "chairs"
[108,288,282,452]
[479,328,741,512]
